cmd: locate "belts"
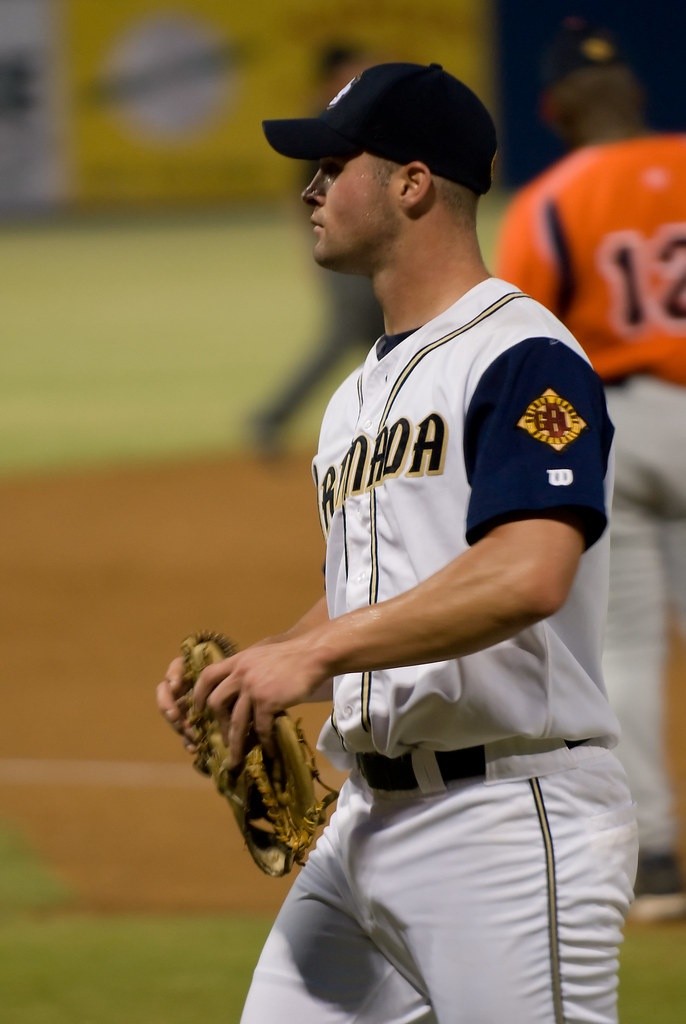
[356,739,591,791]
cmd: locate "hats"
[542,35,630,86]
[262,62,498,196]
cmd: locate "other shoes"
[632,856,686,923]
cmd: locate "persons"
[500,35,686,923]
[248,42,381,451]
[154,62,640,1024]
